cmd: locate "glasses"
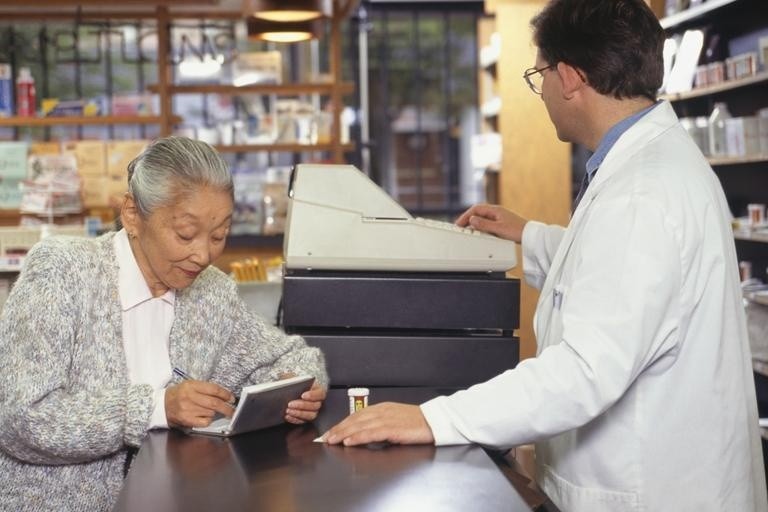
[524,59,585,94]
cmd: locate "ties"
[571,171,590,220]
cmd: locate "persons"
[1,138,330,512]
[322,0,768,512]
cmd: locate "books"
[190,375,316,438]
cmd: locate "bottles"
[740,260,751,281]
[16,65,36,117]
[0,64,16,117]
[707,102,732,156]
[263,165,288,238]
[348,388,370,417]
[746,203,765,226]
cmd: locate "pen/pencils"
[173,367,236,411]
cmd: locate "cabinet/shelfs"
[651,1,768,447]
[1,3,356,280]
